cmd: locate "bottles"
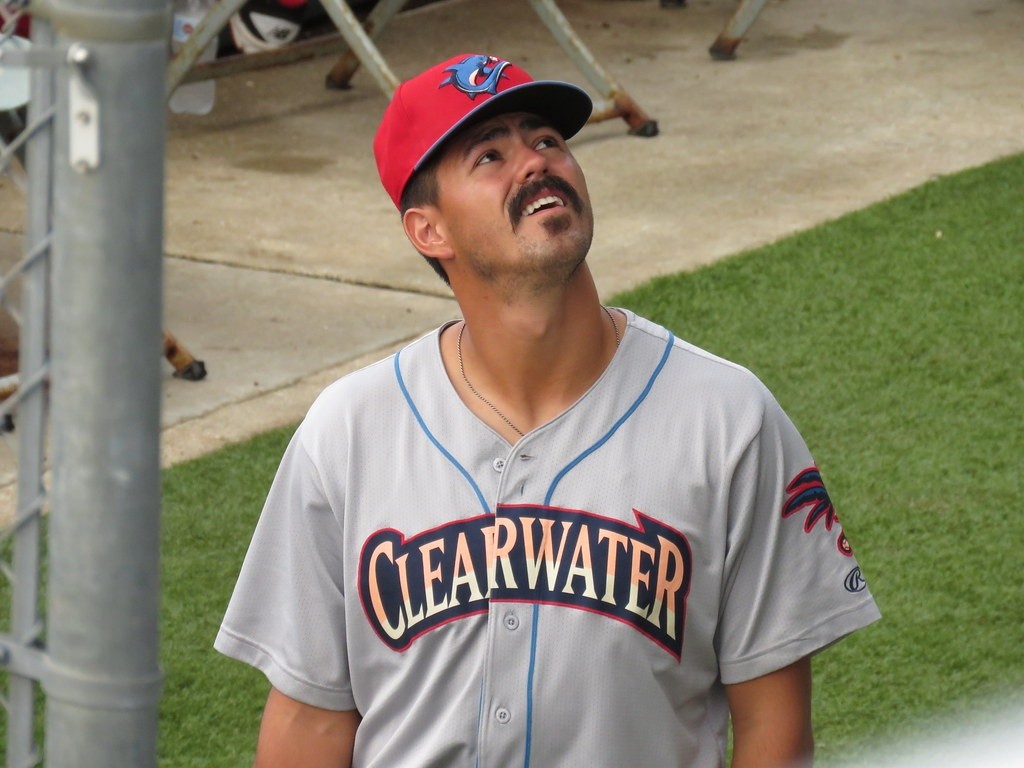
[168,0,218,115]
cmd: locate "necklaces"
[457,303,619,436]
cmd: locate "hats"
[374,54,593,214]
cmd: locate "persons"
[212,52,881,767]
